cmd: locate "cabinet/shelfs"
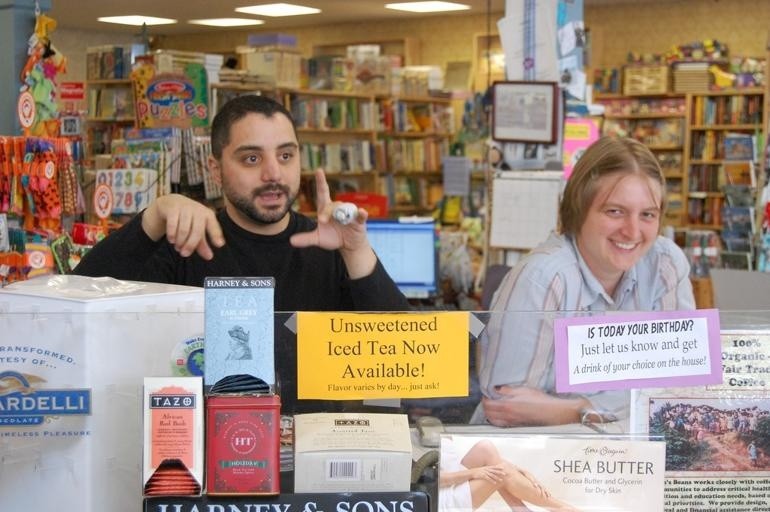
[76,77,138,163]
[685,92,770,235]
[588,96,685,223]
[211,82,455,217]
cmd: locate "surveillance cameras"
[224,54,239,69]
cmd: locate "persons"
[466,133,698,429]
[438,439,589,512]
[649,402,769,470]
[65,95,412,414]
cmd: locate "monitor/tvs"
[365,221,438,300]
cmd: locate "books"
[204,394,282,497]
[142,375,206,498]
[205,274,275,397]
[241,41,454,209]
[686,94,770,272]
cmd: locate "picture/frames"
[493,79,559,144]
